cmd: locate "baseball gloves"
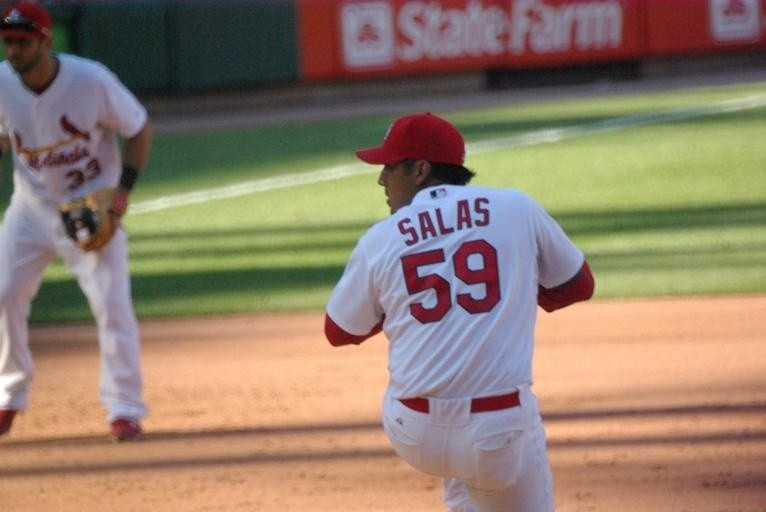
[58,186,129,250]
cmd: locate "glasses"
[1,9,49,36]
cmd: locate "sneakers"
[111,419,139,441]
[0,410,16,435]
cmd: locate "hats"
[357,111,465,165]
[1,2,50,39]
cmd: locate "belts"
[401,391,519,414]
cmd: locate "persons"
[321,110,596,512]
[0,0,151,444]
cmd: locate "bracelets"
[118,161,138,190]
[110,197,126,213]
